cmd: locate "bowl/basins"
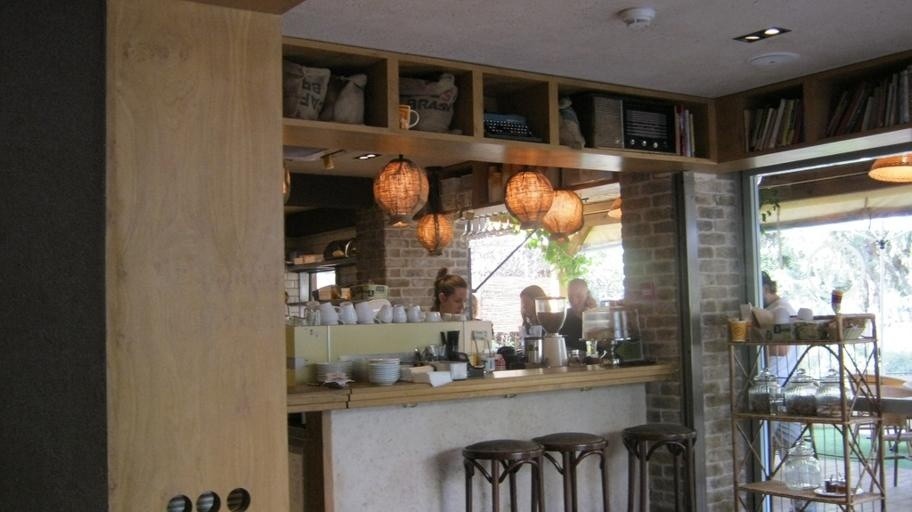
[427,311,441,322]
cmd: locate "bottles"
[782,445,821,491]
[748,366,854,418]
[825,478,846,493]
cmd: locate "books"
[673,96,696,157]
[744,99,802,152]
[826,65,912,136]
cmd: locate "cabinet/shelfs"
[728,337,885,512]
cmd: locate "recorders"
[623,102,675,154]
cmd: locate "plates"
[813,487,863,497]
[317,361,352,383]
[369,357,401,386]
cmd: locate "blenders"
[535,296,569,367]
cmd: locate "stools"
[530,432,609,511]
[463,438,544,512]
[622,422,697,512]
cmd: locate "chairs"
[848,373,912,487]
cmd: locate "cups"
[731,321,746,342]
[399,105,419,130]
[305,302,425,325]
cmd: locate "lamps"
[867,155,912,184]
[541,167,583,241]
[417,166,449,256]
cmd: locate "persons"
[469,295,479,320]
[559,279,597,350]
[762,270,802,461]
[431,268,468,313]
[520,285,550,325]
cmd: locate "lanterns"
[542,190,584,242]
[416,213,453,256]
